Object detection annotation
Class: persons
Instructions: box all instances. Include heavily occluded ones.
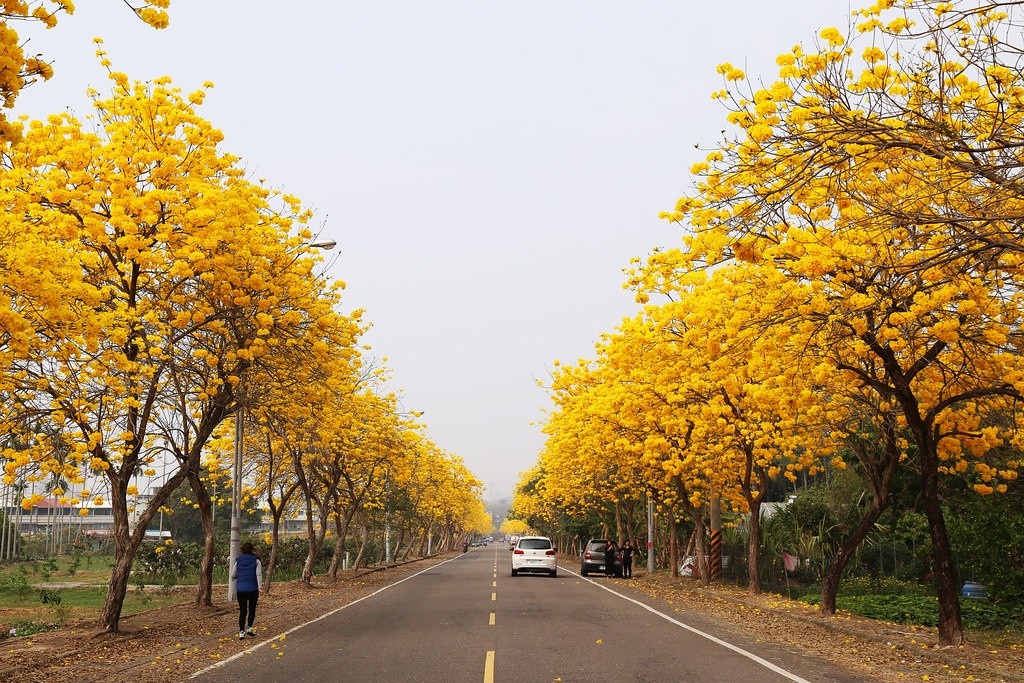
[605,540,615,578]
[464,539,468,548]
[231,542,263,640]
[618,539,635,579]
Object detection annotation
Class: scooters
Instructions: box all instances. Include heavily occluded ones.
[463,545,467,553]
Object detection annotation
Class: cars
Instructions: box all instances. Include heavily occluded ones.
[471,537,494,547]
[498,536,519,551]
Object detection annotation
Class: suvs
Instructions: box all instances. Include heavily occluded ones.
[509,535,559,579]
[578,538,623,578]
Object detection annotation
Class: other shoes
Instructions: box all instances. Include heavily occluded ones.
[239,631,246,640]
[246,627,255,636]
